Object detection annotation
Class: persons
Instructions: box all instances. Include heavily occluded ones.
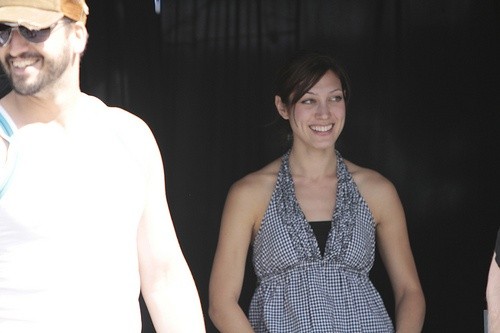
[485,223,500,333]
[0,0,206,333]
[211,57,426,333]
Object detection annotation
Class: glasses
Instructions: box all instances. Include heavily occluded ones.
[0,22,76,48]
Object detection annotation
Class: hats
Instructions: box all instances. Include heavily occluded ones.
[0,0,89,30]
